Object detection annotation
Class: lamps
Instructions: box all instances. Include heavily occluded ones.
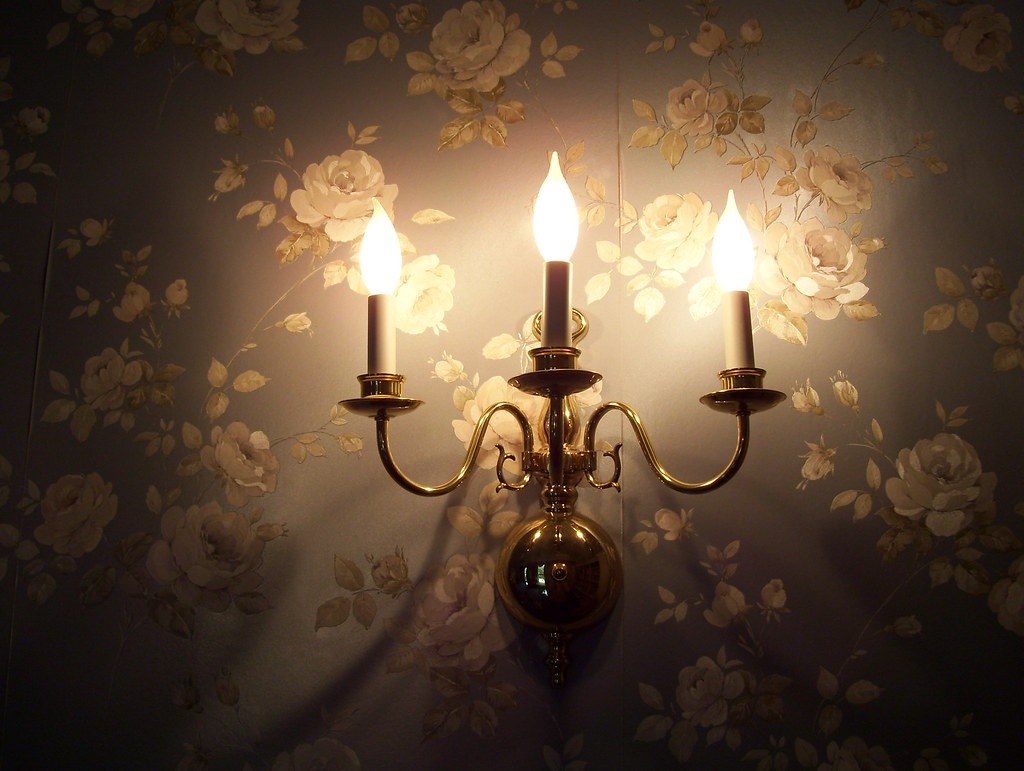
[336,151,786,690]
